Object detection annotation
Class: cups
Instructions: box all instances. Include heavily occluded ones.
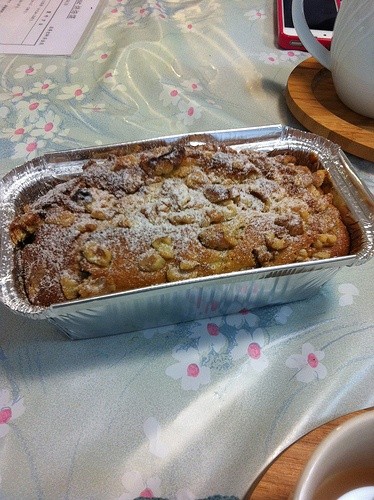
[291,0,374,119]
[291,410,374,500]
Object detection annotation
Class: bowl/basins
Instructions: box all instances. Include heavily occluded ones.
[0,124,374,339]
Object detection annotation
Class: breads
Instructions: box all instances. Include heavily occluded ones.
[10,134,351,305]
